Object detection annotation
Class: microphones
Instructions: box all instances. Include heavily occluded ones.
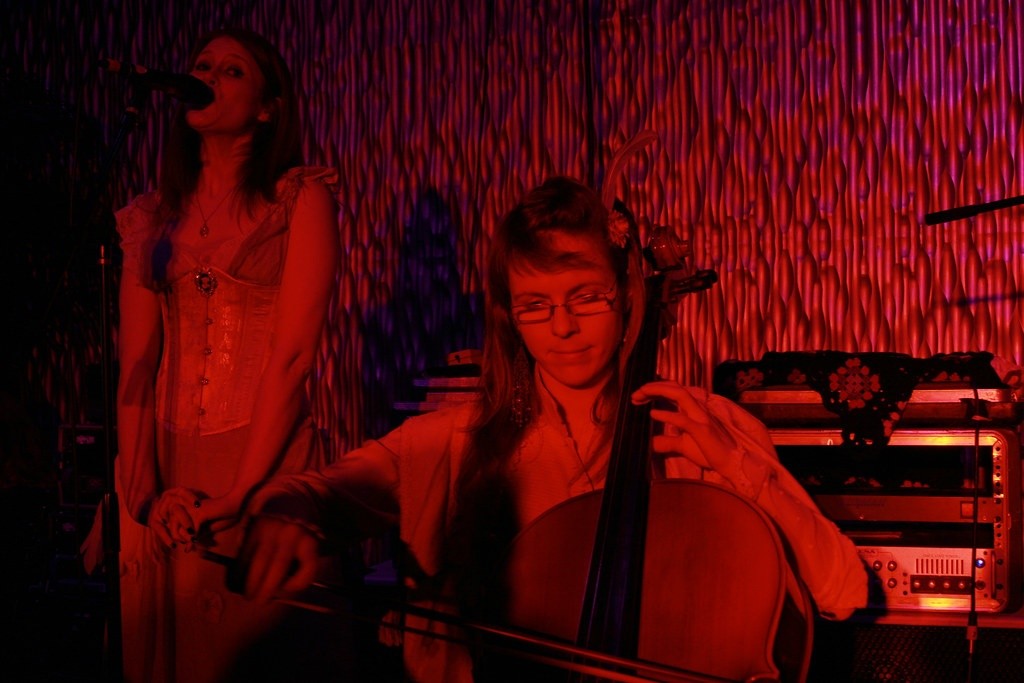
[103,59,214,109]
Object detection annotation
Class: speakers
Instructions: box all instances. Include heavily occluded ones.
[803,607,1024,683]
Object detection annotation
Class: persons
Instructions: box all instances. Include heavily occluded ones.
[237,178,887,682]
[112,32,346,683]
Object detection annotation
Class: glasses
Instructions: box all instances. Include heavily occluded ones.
[506,278,623,324]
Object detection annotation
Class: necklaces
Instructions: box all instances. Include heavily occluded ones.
[191,183,237,239]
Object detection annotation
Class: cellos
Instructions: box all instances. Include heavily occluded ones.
[502,199,813,683]
